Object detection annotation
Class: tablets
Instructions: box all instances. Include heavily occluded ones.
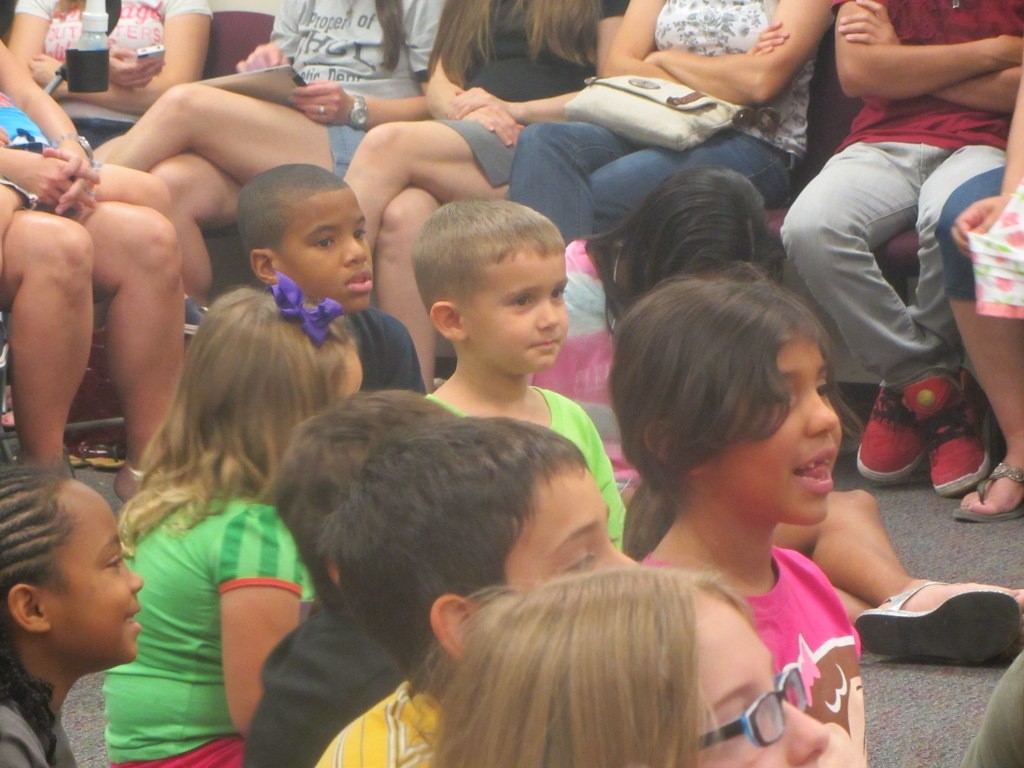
[196,65,308,112]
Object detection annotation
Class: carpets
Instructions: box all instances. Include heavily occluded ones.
[0,392,1023,767]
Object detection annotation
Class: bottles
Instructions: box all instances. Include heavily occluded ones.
[75,11,108,51]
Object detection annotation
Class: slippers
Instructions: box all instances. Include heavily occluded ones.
[952,462,1024,522]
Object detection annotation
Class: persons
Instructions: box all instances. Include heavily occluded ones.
[313,417,635,768]
[104,286,362,768]
[617,262,864,768]
[959,648,1024,768]
[239,391,453,768]
[0,468,145,768]
[430,565,829,768]
[0,0,1024,665]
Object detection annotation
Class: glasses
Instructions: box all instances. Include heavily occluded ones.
[700,662,809,750]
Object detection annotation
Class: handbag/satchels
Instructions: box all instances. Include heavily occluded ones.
[564,75,745,150]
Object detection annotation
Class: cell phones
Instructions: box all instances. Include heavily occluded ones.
[133,45,165,62]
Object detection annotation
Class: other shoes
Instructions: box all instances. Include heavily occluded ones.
[183,298,204,334]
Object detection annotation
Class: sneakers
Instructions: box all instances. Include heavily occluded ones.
[901,370,991,497]
[857,385,929,482]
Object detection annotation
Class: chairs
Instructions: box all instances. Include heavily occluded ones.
[764,202,925,305]
[0,286,200,477]
[205,9,275,80]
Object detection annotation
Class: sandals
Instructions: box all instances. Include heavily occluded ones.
[854,581,1020,661]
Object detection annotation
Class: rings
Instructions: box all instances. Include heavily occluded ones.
[320,106,323,113]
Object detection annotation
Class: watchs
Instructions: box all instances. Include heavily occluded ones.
[78,135,92,158]
[350,95,368,130]
